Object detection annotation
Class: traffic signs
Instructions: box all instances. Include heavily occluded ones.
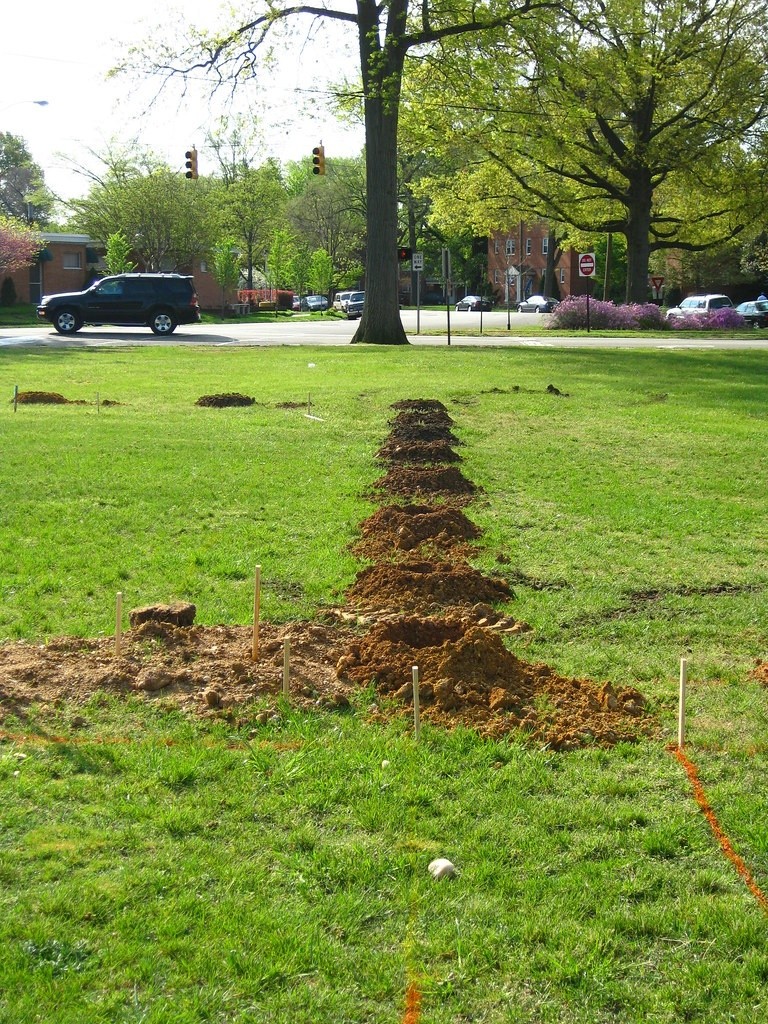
[413,254,424,271]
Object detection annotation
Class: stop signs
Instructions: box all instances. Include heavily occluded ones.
[578,253,596,277]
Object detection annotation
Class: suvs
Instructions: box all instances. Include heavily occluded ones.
[36,272,201,336]
[333,291,364,320]
[665,292,734,321]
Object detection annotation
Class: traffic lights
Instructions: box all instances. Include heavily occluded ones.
[312,139,326,176]
[398,246,411,260]
[184,142,199,180]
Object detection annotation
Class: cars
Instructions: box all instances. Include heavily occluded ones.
[455,295,492,312]
[293,296,300,311]
[301,295,328,312]
[735,301,768,329]
[517,295,560,314]
